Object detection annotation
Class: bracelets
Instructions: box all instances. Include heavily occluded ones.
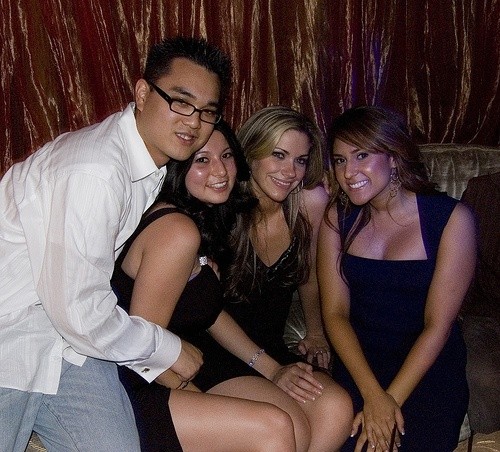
[177,381,188,390]
[249,349,264,367]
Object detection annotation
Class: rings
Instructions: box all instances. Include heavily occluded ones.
[316,350,323,355]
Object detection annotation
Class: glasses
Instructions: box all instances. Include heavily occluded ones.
[149,82,224,124]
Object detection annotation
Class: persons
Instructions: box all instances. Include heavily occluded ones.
[317,106,478,452]
[0,39,333,452]
[112,122,295,452]
[206,106,354,452]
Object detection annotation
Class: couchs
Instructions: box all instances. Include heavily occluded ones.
[283,143,500,452]
